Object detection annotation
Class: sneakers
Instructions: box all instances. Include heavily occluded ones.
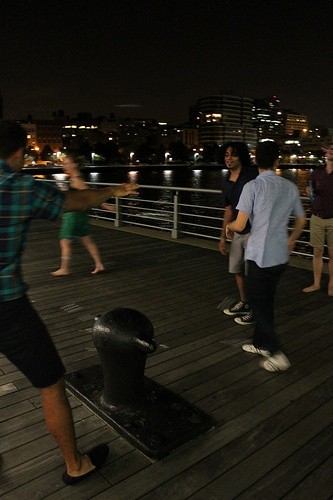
[223,301,250,316]
[264,353,291,372]
[242,344,271,357]
[235,312,256,325]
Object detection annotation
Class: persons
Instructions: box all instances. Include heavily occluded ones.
[218,142,259,326]
[0,117,139,483]
[225,140,307,373]
[49,156,105,277]
[301,144,333,298]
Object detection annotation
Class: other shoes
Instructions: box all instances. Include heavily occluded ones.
[62,443,109,485]
